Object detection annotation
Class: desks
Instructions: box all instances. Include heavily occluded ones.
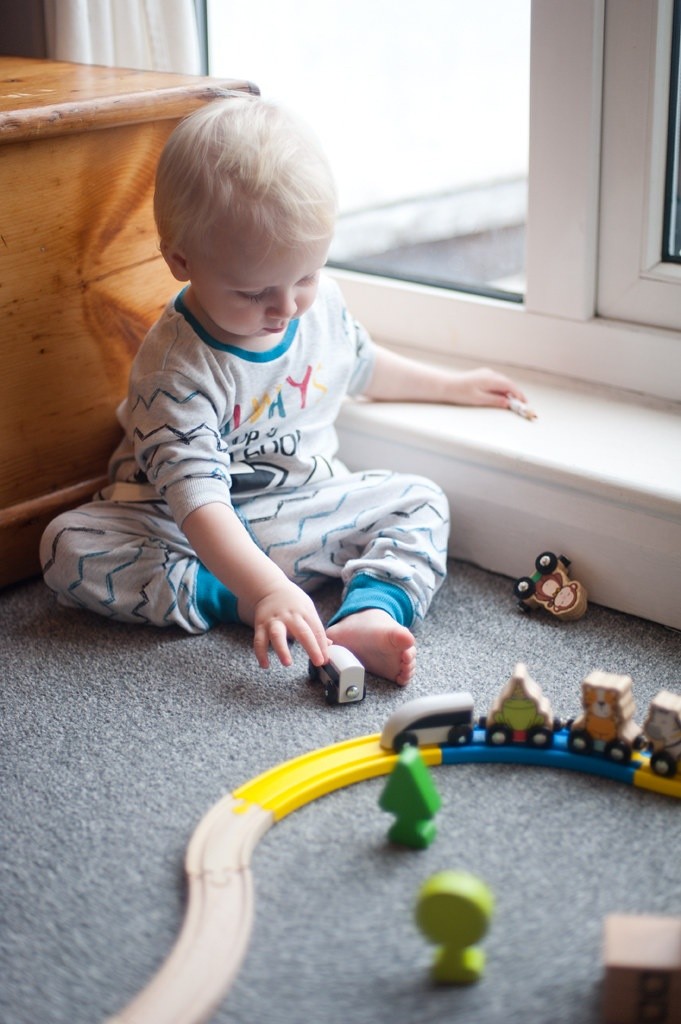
[0,49,259,582]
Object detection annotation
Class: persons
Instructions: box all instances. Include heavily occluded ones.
[37,82,530,689]
